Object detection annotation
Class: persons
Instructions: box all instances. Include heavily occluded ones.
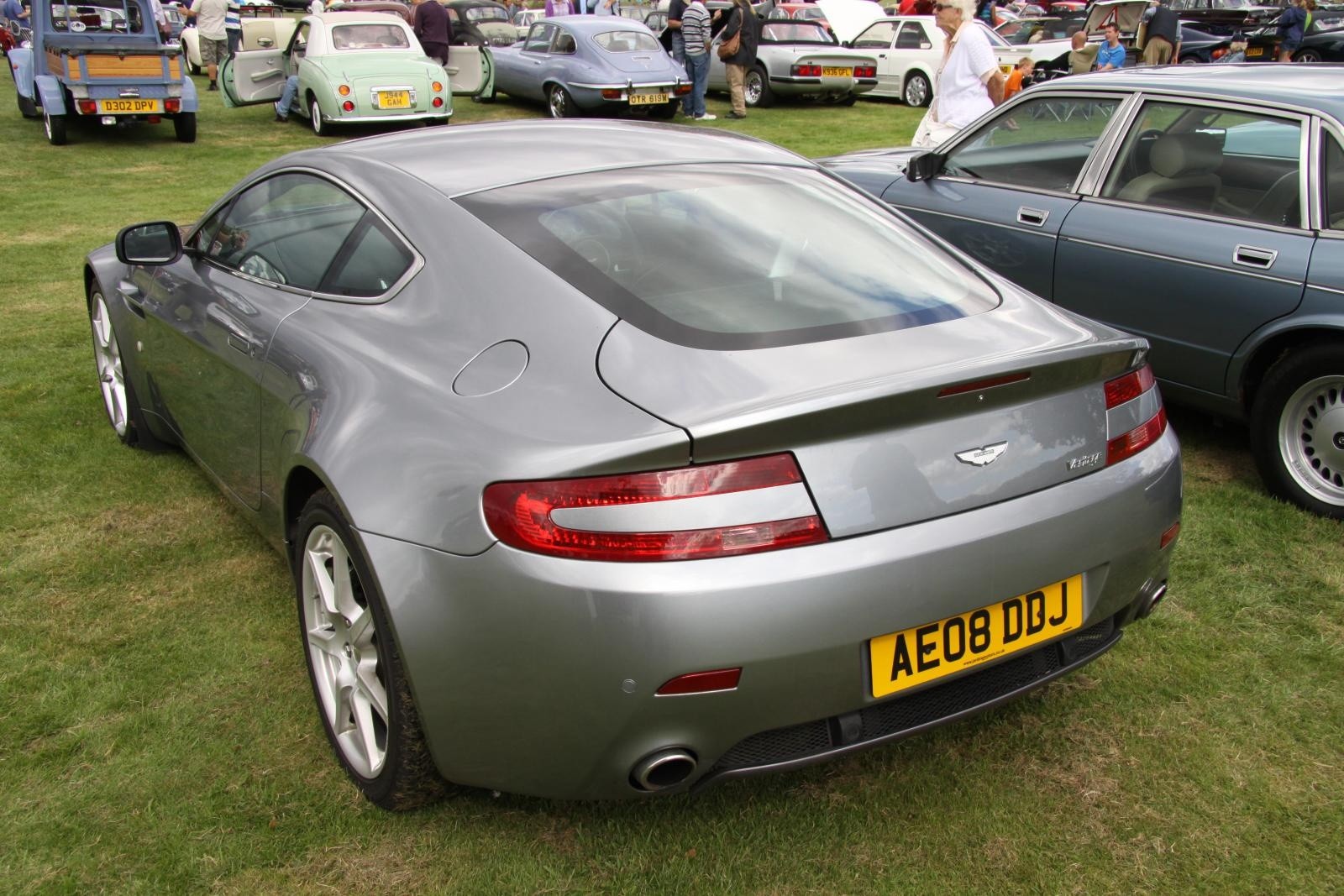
[1303,0,1317,33]
[501,0,534,25]
[1095,21,1125,71]
[587,0,617,15]
[1277,0,1311,62]
[414,0,454,67]
[545,0,575,40]
[273,75,298,121]
[1021,30,1087,117]
[975,0,997,29]
[911,0,1020,179]
[897,0,936,29]
[177,0,234,89]
[4,0,32,29]
[311,0,323,15]
[1142,1,1183,65]
[1211,33,1248,63]
[573,0,594,14]
[667,0,759,120]
[1004,57,1035,102]
[151,0,166,33]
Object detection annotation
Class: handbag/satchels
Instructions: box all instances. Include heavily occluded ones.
[910,101,959,150]
[717,8,744,56]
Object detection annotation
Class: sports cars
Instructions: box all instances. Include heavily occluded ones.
[80,116,1190,812]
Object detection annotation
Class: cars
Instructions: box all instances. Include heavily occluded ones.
[482,13,695,118]
[511,8,546,39]
[803,59,1343,527]
[156,0,523,138]
[617,6,669,31]
[637,0,1344,107]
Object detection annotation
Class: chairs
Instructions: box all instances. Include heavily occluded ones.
[1247,131,1344,228]
[557,34,572,49]
[1026,67,1116,123]
[1066,26,1081,37]
[897,32,921,49]
[377,35,400,46]
[112,19,141,33]
[341,30,370,47]
[607,40,628,52]
[1027,29,1053,45]
[1115,132,1222,214]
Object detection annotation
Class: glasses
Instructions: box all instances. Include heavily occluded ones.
[933,3,952,11]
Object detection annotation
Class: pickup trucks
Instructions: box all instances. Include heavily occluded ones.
[7,0,200,143]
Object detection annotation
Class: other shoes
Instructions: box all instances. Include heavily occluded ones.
[695,112,717,120]
[23,111,41,121]
[685,114,694,118]
[725,112,746,119]
[729,110,734,113]
[208,85,218,91]
[274,102,288,123]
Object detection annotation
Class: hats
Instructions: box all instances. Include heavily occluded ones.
[1231,33,1245,42]
